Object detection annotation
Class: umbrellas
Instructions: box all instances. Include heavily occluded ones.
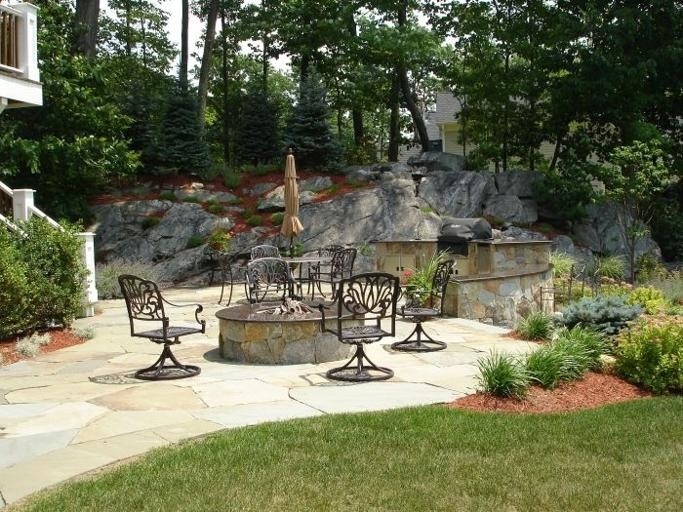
[279,146,304,260]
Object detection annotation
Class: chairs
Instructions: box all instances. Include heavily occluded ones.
[206,246,458,382]
[116,275,206,380]
